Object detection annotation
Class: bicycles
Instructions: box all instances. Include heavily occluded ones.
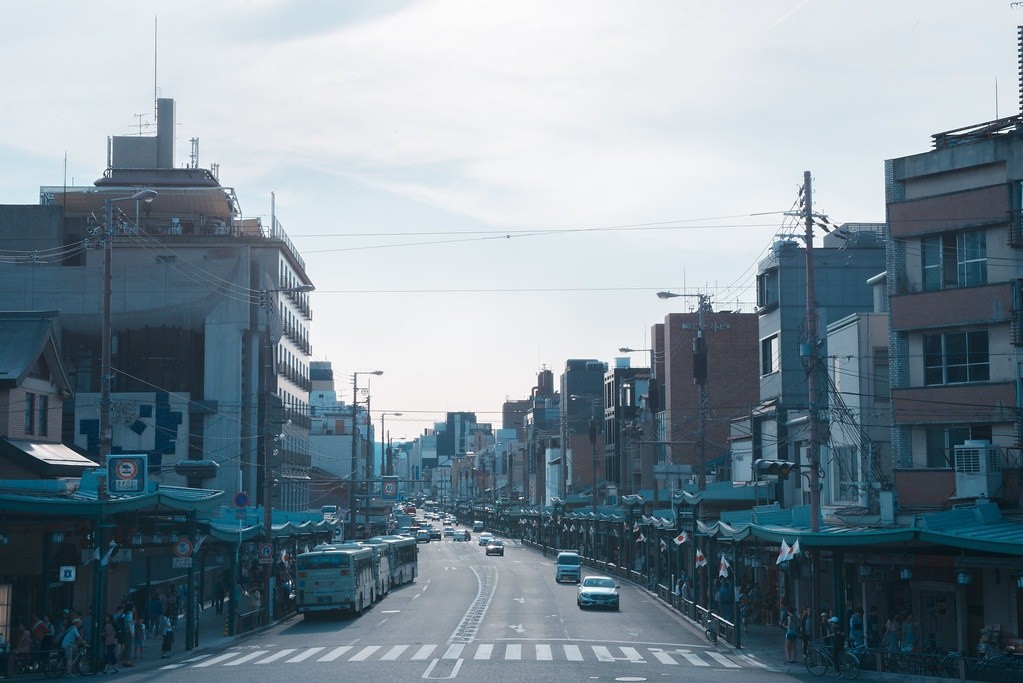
[805,636,861,680]
[702,610,718,646]
[43,643,102,679]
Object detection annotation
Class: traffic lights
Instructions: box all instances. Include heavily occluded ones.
[754,459,797,480]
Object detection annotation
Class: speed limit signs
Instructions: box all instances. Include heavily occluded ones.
[383,482,397,495]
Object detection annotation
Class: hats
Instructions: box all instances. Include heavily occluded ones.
[829,617,838,622]
[62,609,69,614]
[821,613,828,617]
[72,618,84,624]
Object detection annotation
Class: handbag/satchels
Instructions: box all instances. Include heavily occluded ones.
[58,635,65,648]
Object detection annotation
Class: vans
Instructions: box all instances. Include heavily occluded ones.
[554,552,583,584]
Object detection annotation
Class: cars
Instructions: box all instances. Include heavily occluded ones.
[401,502,505,556]
[577,576,620,611]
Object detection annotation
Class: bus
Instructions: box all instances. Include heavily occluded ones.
[295,535,420,621]
[423,501,438,513]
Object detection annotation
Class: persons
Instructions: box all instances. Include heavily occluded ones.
[713,576,773,632]
[101,583,181,674]
[0,608,87,677]
[676,569,693,603]
[215,561,291,627]
[819,609,844,679]
[849,607,865,648]
[633,555,662,591]
[580,542,584,557]
[881,609,915,671]
[866,605,881,649]
[783,606,810,664]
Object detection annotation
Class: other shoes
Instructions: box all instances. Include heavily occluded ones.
[66,672,76,678]
[114,668,119,672]
[784,661,791,665]
[835,673,843,679]
[29,666,33,670]
[34,664,39,669]
[790,660,796,664]
[101,670,107,674]
[161,655,171,659]
[123,663,135,667]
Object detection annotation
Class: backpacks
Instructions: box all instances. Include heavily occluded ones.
[118,612,132,632]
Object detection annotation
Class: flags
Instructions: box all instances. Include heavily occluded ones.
[695,549,707,568]
[624,522,645,542]
[562,524,594,534]
[304,546,309,552]
[673,530,689,545]
[281,550,288,568]
[719,557,730,578]
[776,538,800,565]
[192,535,207,553]
[519,517,562,528]
[83,548,100,567]
[660,540,666,552]
[101,547,114,566]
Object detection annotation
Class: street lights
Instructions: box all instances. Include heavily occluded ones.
[619,348,659,510]
[390,438,406,446]
[570,395,596,515]
[498,442,512,491]
[382,413,403,476]
[523,427,540,503]
[351,371,383,539]
[657,292,707,491]
[262,284,314,624]
[89,190,158,672]
